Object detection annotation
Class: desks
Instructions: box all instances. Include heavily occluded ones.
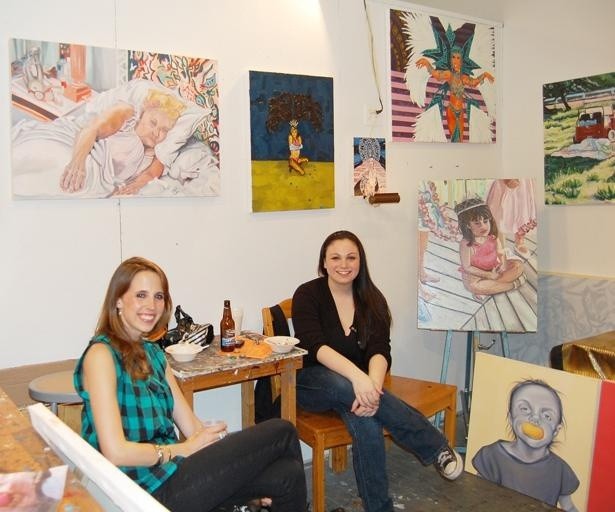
[560,331,615,382]
[27,367,81,415]
[164,329,304,441]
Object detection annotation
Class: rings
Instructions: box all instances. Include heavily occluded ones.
[219,433,226,440]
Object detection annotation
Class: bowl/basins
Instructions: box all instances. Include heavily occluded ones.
[264,335,300,353]
[164,343,203,362]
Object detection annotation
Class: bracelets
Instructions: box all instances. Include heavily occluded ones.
[166,446,172,462]
[154,444,164,468]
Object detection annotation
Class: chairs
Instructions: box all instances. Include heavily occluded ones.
[382,369,456,453]
[260,296,386,511]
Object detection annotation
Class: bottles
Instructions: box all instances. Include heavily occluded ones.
[219,300,236,351]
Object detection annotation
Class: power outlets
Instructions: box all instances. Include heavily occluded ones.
[364,105,381,125]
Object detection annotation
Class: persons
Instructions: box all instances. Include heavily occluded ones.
[60,89,188,196]
[453,198,528,302]
[418,182,458,301]
[471,380,579,512]
[288,126,308,175]
[291,231,463,512]
[487,179,537,253]
[72,257,343,512]
[416,46,495,142]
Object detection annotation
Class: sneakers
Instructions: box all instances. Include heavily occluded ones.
[434,444,464,480]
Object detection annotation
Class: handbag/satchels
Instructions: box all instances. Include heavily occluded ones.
[254,375,281,422]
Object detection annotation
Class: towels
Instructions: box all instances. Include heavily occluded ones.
[216,335,273,359]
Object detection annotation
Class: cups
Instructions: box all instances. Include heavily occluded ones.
[232,308,243,337]
